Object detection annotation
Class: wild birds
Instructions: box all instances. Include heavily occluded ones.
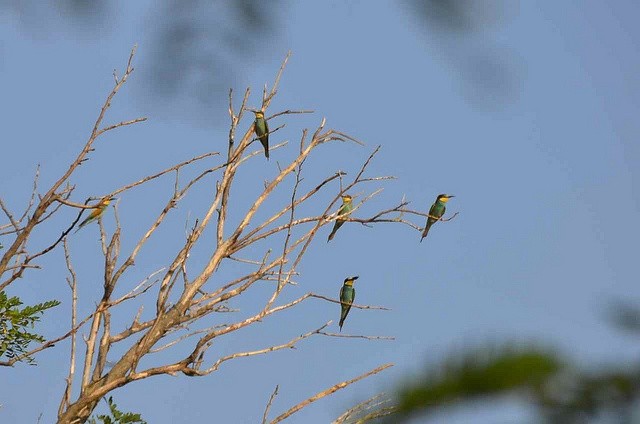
[327,195,352,243]
[251,110,270,160]
[339,276,358,332]
[74,196,117,235]
[420,193,456,243]
